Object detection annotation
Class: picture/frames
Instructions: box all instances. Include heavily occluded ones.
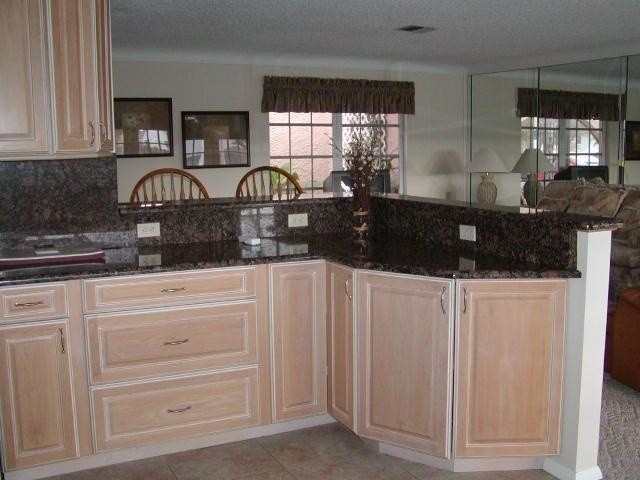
[181,110,251,169]
[113,97,174,158]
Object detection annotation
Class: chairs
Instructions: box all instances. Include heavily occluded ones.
[236,165,303,198]
[130,168,209,203]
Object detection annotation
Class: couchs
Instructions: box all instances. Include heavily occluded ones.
[535,181,640,392]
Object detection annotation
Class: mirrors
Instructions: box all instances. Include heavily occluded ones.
[467,54,640,209]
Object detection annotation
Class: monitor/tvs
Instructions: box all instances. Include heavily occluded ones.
[554,165,609,184]
[323,169,391,193]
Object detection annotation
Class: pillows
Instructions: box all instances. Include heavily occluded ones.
[533,181,640,245]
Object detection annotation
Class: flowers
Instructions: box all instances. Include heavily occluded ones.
[322,109,398,234]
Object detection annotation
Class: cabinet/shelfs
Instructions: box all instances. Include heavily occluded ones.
[357,269,567,459]
[270,261,328,423]
[1,283,74,473]
[329,263,357,436]
[0,0,116,163]
[81,265,261,455]
